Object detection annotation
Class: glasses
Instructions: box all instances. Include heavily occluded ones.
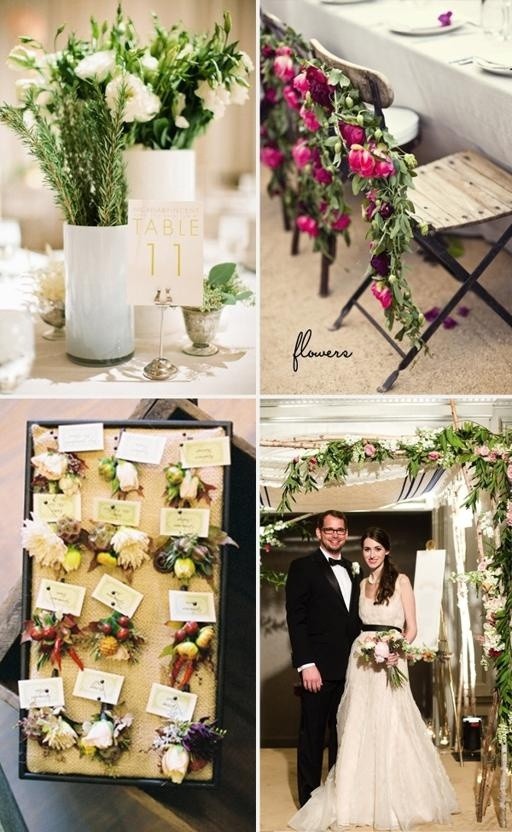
[324,528,346,535]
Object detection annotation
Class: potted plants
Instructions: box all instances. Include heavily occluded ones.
[180,262,253,358]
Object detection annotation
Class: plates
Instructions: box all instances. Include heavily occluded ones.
[320,0,512,81]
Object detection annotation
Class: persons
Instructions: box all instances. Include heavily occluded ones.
[286,510,364,808]
[345,527,418,831]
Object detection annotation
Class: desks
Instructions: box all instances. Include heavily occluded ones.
[261,1,511,252]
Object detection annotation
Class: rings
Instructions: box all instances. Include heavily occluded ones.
[394,662,396,666]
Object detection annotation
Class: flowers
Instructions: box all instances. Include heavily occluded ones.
[355,626,410,688]
[65,5,257,149]
[2,29,159,226]
[260,511,290,552]
[277,419,511,724]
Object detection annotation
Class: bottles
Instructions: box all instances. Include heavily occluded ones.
[0,216,38,393]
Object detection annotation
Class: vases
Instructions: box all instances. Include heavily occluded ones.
[121,143,194,311]
[61,221,136,366]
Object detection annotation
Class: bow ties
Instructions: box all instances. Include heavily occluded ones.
[329,558,347,568]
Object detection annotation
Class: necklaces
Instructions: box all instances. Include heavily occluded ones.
[368,573,382,585]
